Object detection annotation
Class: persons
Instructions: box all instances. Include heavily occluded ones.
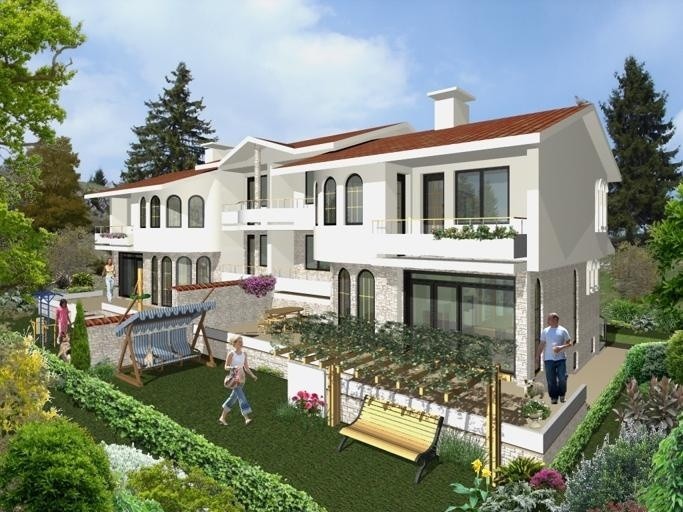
[101,257,116,303]
[53,299,72,345]
[218,334,258,427]
[535,312,573,404]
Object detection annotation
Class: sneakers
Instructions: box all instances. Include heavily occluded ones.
[218,418,229,426]
[560,395,566,403]
[245,418,254,426]
[551,395,558,404]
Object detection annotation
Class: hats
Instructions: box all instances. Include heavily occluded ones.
[227,333,241,346]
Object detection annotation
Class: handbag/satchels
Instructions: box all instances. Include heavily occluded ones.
[222,367,241,390]
